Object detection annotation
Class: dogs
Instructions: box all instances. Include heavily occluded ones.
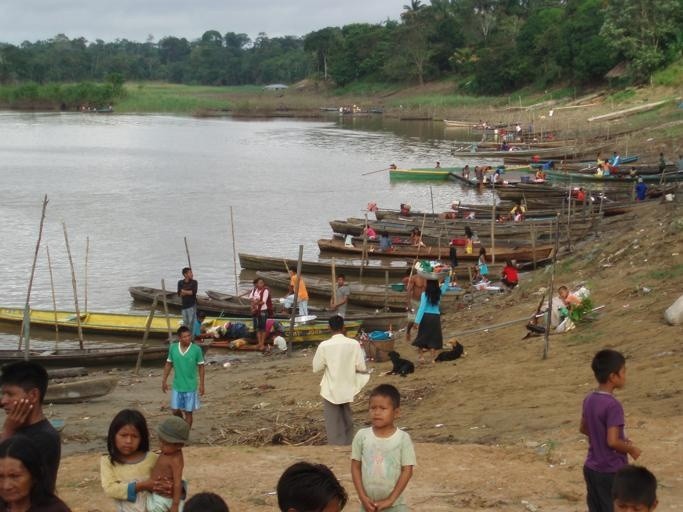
[435,340,463,362]
[386,351,415,377]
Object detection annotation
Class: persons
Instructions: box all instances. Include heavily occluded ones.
[277,461,348,512]
[462,225,518,291]
[658,152,665,172]
[270,332,288,354]
[611,464,657,512]
[178,267,198,332]
[535,161,556,180]
[463,165,503,184]
[363,202,423,251]
[559,285,592,322]
[288,266,309,316]
[330,275,350,320]
[101,409,188,512]
[183,492,229,512]
[312,315,370,445]
[479,120,553,151]
[162,326,205,429]
[248,278,271,351]
[578,187,586,199]
[511,201,525,224]
[403,268,450,362]
[596,152,618,175]
[146,416,190,512]
[351,384,416,512]
[0,361,61,479]
[0,434,72,512]
[578,350,641,512]
[630,167,647,201]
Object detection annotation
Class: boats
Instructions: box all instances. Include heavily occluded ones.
[204,290,408,330]
[127,286,403,332]
[237,252,516,279]
[0,343,209,366]
[255,271,466,311]
[80,109,113,114]
[319,108,382,114]
[42,376,119,404]
[0,307,363,343]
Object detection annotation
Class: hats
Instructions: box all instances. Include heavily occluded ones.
[153,416,190,446]
